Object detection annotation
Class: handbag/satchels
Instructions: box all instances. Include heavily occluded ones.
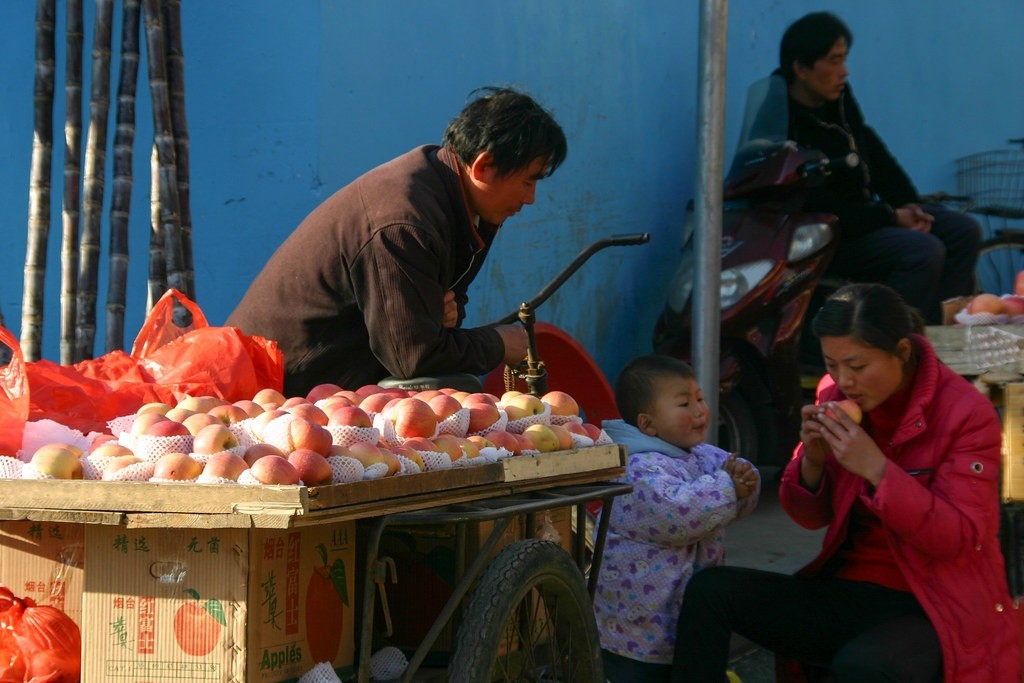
[0,326,30,457]
[131,288,285,403]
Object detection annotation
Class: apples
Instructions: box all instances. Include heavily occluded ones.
[0,599,83,683]
[966,269,1024,317]
[825,399,863,426]
[28,384,602,487]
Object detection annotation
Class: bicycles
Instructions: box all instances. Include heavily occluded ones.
[945,137,1024,296]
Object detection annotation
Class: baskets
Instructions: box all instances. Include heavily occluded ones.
[954,149,1024,217]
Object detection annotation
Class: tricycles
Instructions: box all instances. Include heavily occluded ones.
[0,232,652,683]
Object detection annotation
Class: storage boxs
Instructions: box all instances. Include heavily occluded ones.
[0,520,85,630]
[988,382,1024,504]
[355,506,572,658]
[80,520,357,683]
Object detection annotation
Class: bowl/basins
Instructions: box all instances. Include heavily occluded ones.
[481,321,621,517]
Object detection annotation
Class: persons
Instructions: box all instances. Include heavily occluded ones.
[225,86,569,398]
[775,281,1024,683]
[591,355,760,683]
[751,11,982,324]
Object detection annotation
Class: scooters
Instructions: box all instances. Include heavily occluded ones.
[650,71,917,498]
